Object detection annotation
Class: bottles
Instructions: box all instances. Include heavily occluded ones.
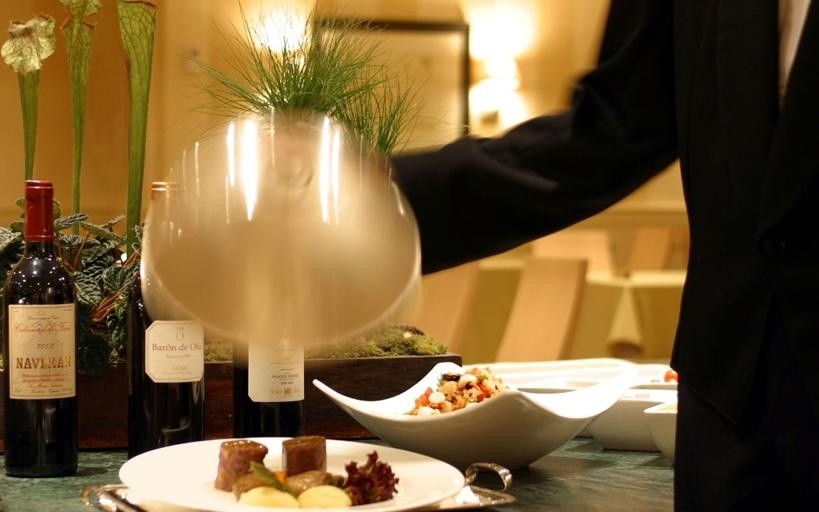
[2,179,82,479]
[230,334,308,439]
[126,179,206,461]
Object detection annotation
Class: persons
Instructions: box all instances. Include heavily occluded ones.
[387,0,818,512]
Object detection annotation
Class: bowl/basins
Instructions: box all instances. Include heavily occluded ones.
[310,359,635,473]
[520,384,678,463]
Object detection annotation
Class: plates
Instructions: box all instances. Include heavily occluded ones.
[115,434,471,512]
